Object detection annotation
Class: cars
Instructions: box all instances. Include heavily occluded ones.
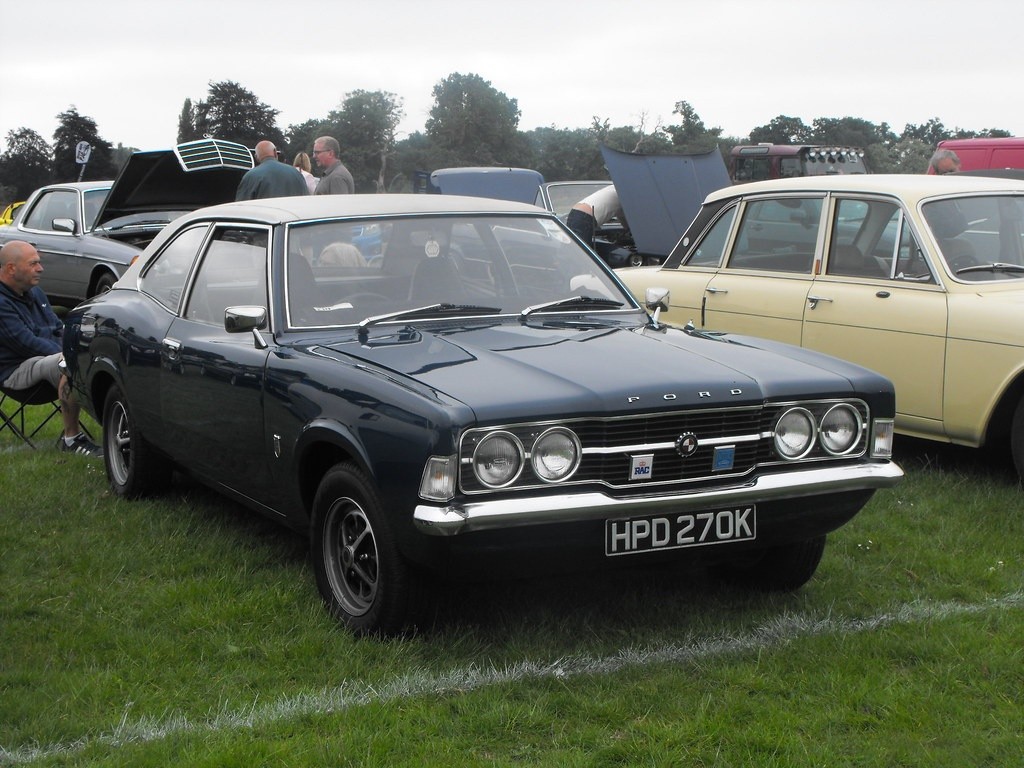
[925,135,1024,262]
[1,139,254,310]
[62,195,907,637]
[596,140,1024,483]
[426,161,639,267]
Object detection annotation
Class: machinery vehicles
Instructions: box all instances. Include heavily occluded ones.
[727,134,916,261]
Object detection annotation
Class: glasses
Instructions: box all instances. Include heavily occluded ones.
[313,150,331,155]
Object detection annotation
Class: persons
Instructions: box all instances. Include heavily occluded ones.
[0,240,105,459]
[318,243,367,267]
[313,136,354,259]
[292,152,316,195]
[235,140,311,265]
[931,150,962,175]
[567,185,629,248]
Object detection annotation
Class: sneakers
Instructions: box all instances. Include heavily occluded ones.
[62,432,104,458]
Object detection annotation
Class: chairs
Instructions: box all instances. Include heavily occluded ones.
[828,244,879,278]
[261,252,339,329]
[407,253,473,311]
[0,378,96,451]
[936,236,980,282]
